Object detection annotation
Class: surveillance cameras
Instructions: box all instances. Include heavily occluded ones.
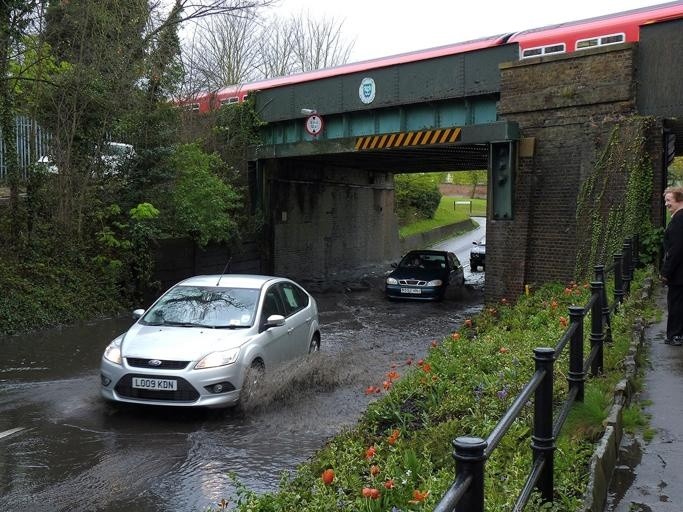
[301,109,316,115]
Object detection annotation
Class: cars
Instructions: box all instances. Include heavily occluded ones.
[97,272,325,415]
[383,248,466,302]
[48,139,140,181]
[468,233,486,272]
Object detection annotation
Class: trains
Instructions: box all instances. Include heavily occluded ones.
[170,0,683,120]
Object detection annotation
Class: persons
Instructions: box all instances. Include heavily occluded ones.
[657,185,683,348]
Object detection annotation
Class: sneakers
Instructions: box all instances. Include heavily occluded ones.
[665,336,683,345]
